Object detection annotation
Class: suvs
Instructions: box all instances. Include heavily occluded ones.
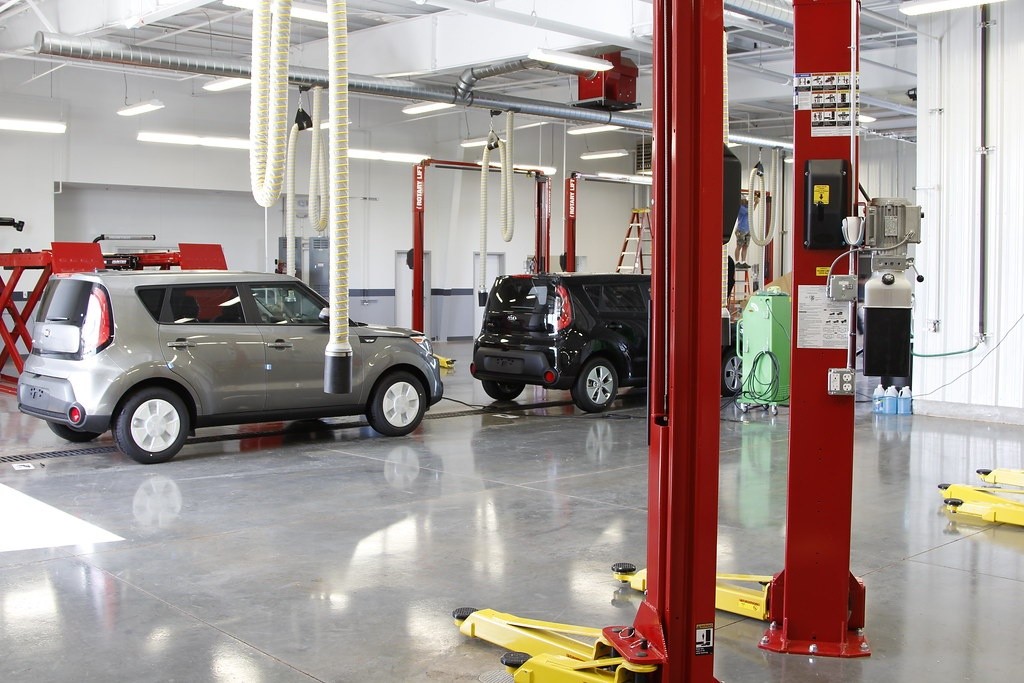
[16,269,444,464]
[469,271,742,412]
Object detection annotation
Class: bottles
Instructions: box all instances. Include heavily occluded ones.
[872,384,912,414]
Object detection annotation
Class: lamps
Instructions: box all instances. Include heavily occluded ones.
[727,131,796,153]
[480,161,555,175]
[0,54,434,171]
[599,173,652,186]
[567,124,623,135]
[224,0,330,24]
[462,136,499,148]
[403,100,453,115]
[528,48,612,72]
[581,149,628,159]
[897,0,1006,17]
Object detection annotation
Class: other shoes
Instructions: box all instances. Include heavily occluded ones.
[743,263,751,268]
[736,262,743,268]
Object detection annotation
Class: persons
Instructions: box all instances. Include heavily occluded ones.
[735,194,761,269]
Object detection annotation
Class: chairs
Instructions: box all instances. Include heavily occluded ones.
[183,294,199,324]
[216,299,245,323]
